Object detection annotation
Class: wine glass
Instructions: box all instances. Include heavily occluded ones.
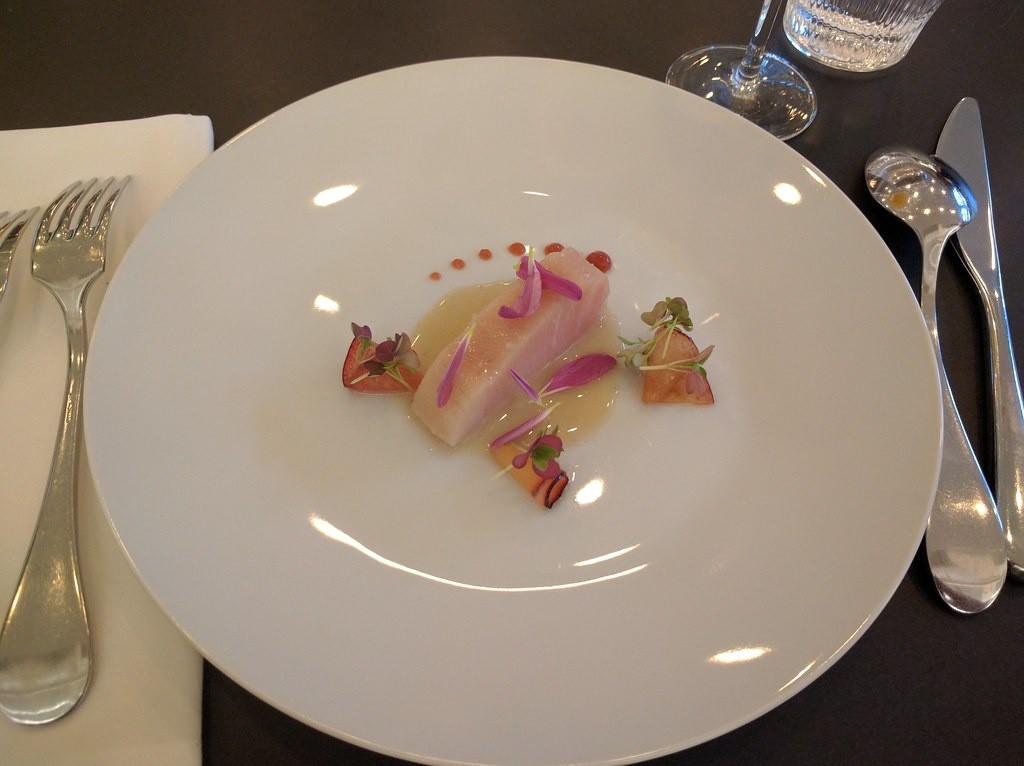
[665,0,818,141]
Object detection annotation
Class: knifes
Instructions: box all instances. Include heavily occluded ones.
[936,96,1024,580]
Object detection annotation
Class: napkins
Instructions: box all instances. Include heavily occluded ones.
[0,110,216,766]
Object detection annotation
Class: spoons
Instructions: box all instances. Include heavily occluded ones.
[865,143,1009,614]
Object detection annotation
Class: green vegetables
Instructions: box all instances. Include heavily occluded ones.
[351,245,714,482]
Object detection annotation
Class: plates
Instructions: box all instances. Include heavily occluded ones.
[86,55,942,766]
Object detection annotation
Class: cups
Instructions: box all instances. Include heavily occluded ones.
[782,0,944,73]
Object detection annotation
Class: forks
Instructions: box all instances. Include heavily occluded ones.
[0,176,131,725]
[0,206,40,302]
